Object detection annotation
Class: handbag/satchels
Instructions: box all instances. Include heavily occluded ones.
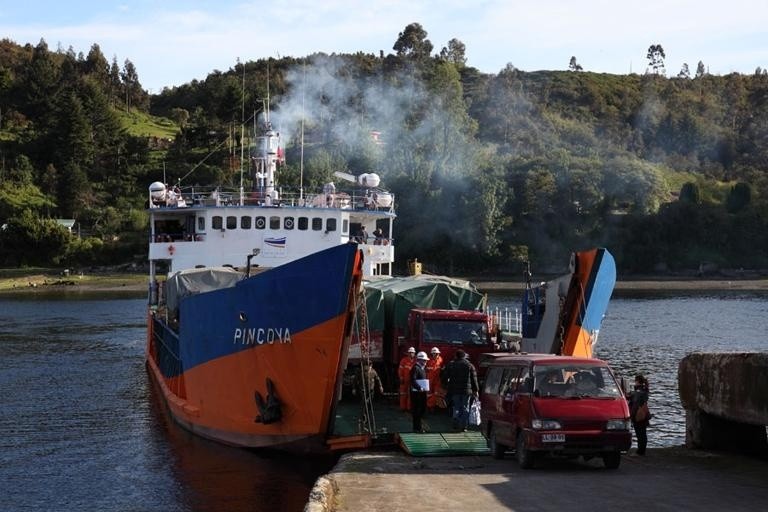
[468,401,482,425]
[635,402,649,422]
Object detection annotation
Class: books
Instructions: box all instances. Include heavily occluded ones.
[412,378,430,392]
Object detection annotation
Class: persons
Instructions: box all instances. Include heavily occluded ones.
[408,350,431,432]
[442,348,480,431]
[352,359,384,425]
[355,225,369,243]
[425,346,445,412]
[624,374,652,456]
[322,177,336,208]
[490,323,503,345]
[397,347,417,417]
[155,229,195,242]
[372,228,385,245]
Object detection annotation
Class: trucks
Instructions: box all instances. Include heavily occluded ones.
[353,272,497,402]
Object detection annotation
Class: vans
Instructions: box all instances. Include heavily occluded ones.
[479,353,633,473]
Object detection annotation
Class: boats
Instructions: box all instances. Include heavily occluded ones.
[146,59,618,450]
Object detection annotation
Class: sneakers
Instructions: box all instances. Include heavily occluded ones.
[453,422,471,432]
[430,409,447,415]
[399,409,413,414]
[416,429,426,433]
[630,451,646,457]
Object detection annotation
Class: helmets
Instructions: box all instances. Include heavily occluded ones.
[417,352,430,360]
[430,347,441,353]
[408,347,416,352]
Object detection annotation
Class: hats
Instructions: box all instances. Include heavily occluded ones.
[365,360,372,366]
[374,228,385,238]
[456,351,465,357]
